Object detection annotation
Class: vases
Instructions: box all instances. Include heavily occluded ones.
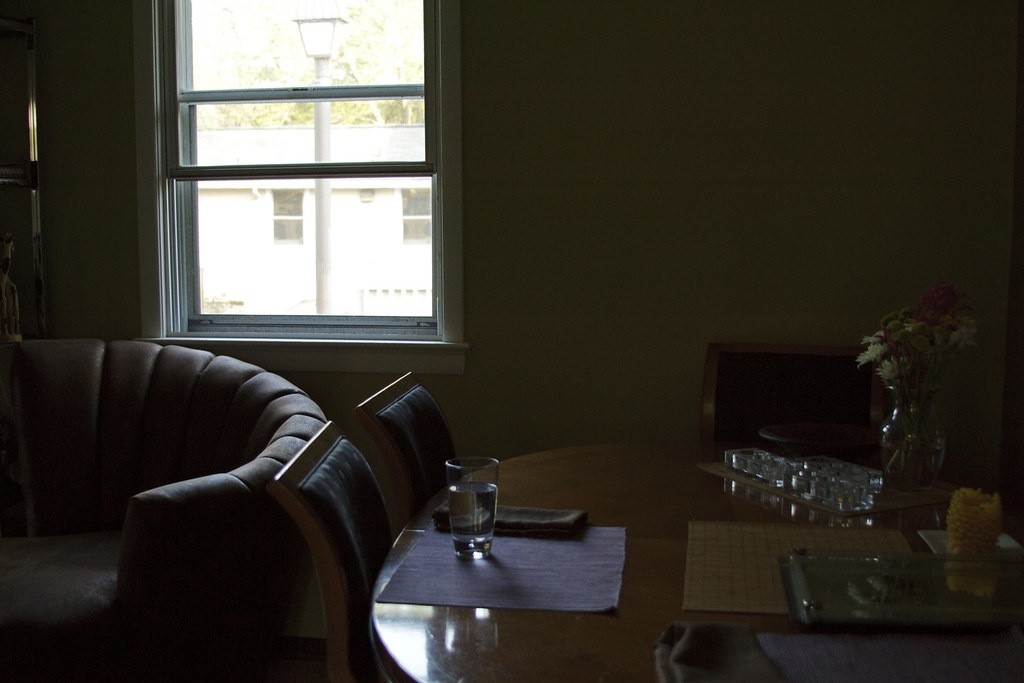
[880,400,942,492]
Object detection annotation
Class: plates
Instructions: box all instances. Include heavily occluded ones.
[916,528,1024,554]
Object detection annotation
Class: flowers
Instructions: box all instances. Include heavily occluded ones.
[857,279,977,469]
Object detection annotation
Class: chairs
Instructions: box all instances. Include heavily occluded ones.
[352,372,462,525]
[267,420,392,683]
[698,343,885,441]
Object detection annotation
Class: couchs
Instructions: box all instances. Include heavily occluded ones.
[0,337,328,683]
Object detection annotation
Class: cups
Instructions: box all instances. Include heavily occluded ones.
[445,456,500,560]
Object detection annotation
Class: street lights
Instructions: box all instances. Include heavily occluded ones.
[291,0,351,315]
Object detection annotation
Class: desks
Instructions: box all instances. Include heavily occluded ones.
[368,435,1024,683]
